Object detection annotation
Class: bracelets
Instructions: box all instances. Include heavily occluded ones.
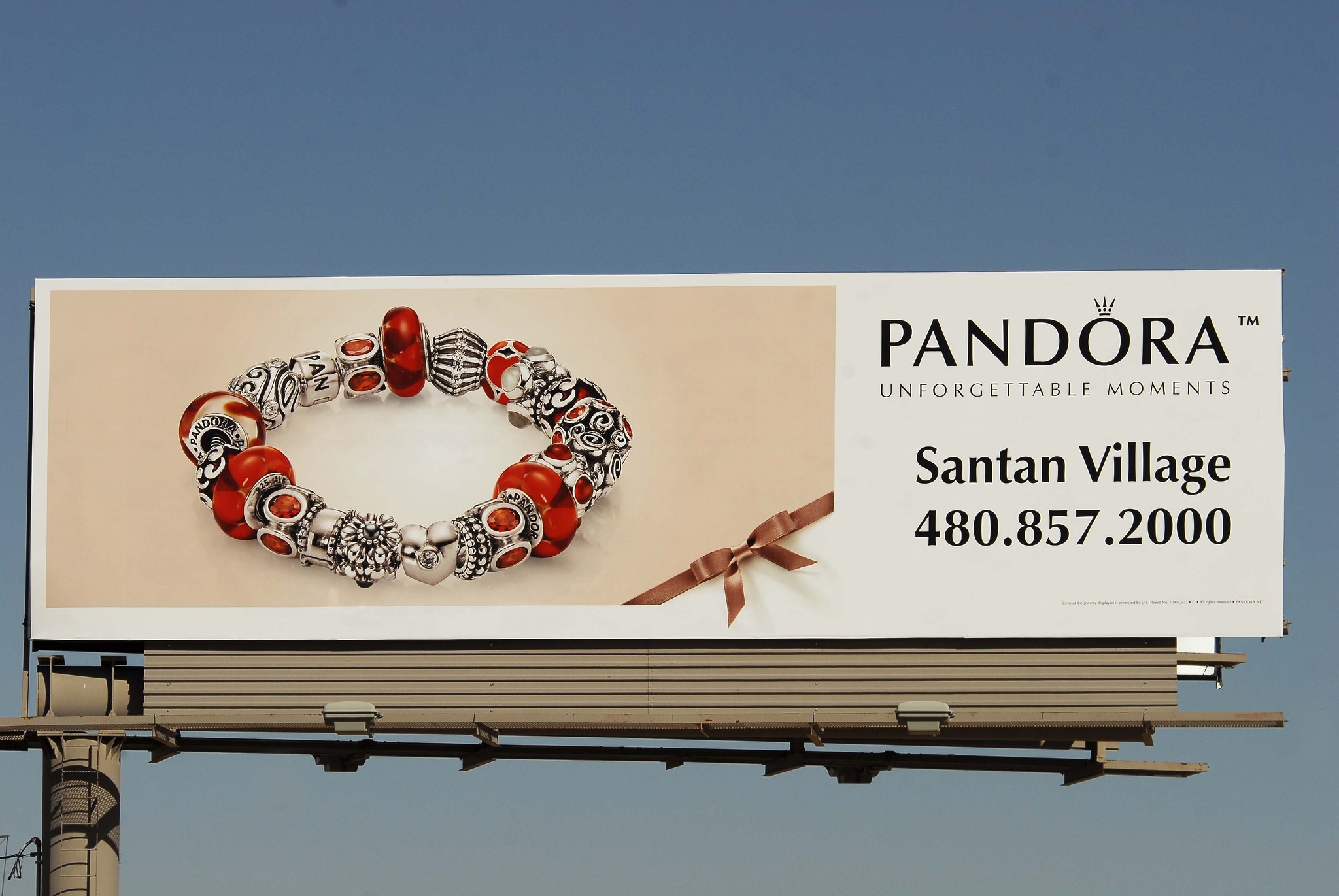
[179,307,634,589]
